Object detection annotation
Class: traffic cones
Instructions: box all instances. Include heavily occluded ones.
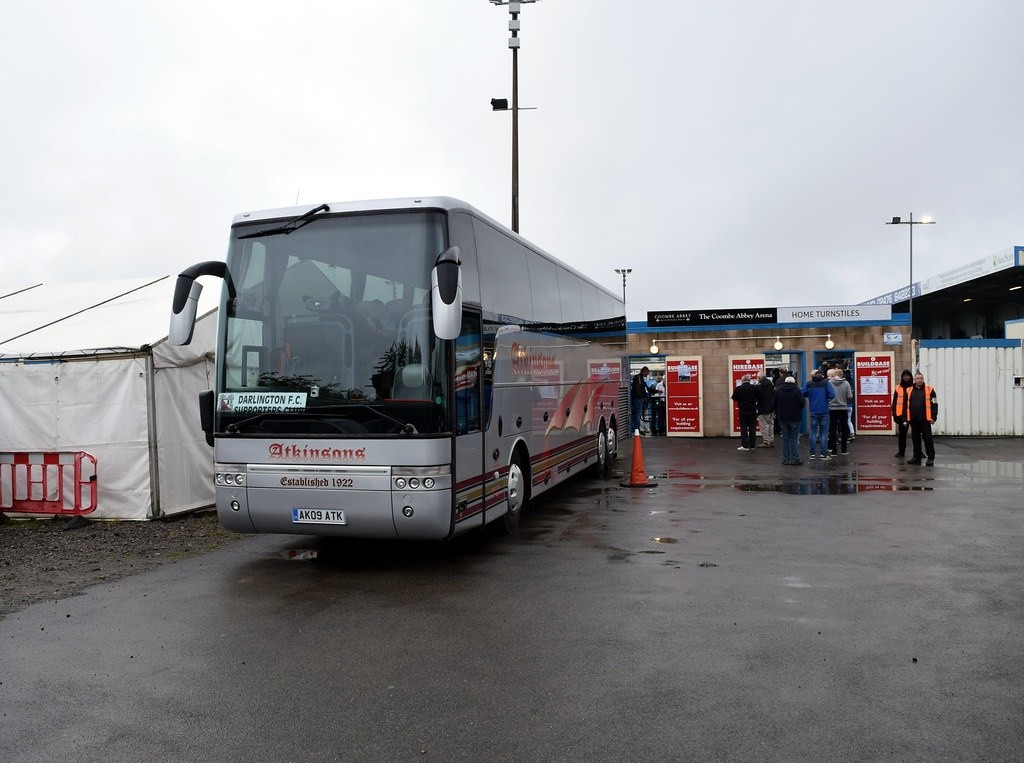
[617,427,660,490]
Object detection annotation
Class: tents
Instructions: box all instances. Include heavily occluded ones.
[0,275,224,519]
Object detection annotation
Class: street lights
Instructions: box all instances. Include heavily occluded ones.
[488,0,542,234]
[884,212,939,339]
[614,268,632,304]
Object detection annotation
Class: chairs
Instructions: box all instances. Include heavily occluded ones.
[318,288,432,401]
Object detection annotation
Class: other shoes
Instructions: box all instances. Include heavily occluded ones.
[895,452,905,457]
[639,431,646,435]
[770,444,775,448]
[926,459,934,466]
[758,443,769,448]
[907,457,921,465]
[922,453,926,458]
[841,450,849,455]
[838,434,855,444]
[830,453,836,457]
[782,460,790,465]
[737,446,750,450]
[791,460,805,465]
[827,448,832,452]
[809,454,816,460]
[751,447,756,451]
[820,455,832,461]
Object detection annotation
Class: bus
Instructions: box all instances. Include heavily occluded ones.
[165,196,633,552]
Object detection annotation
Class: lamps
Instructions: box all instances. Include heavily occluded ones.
[825,333,834,350]
[774,336,783,350]
[649,340,658,354]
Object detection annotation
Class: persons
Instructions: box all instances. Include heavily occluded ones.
[771,368,806,464]
[731,375,756,451]
[802,358,856,460]
[755,372,775,447]
[632,366,663,434]
[892,370,926,458]
[648,374,666,436]
[903,373,938,466]
